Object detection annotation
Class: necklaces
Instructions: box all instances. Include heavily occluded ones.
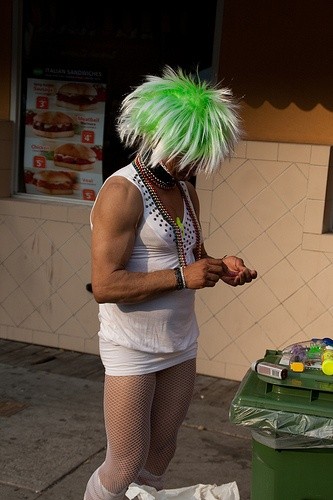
[135,145,202,269]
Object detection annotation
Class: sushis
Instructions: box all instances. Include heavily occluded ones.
[32,111,75,139]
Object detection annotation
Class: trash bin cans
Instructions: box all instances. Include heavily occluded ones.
[227,350,332,500]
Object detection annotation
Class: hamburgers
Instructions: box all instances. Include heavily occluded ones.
[55,83,99,111]
[53,143,96,171]
[32,171,81,195]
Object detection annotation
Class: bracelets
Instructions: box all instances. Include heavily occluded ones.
[173,267,183,290]
[178,266,185,289]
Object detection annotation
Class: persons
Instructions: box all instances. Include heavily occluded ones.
[80,67,259,500]
